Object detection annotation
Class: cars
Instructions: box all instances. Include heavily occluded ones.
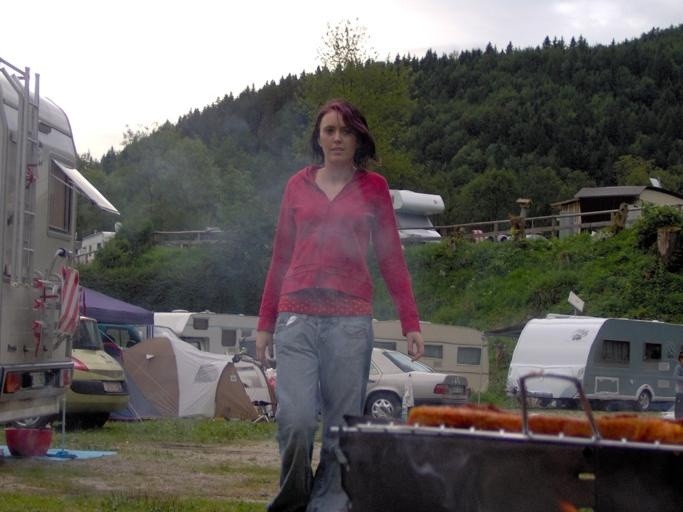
[259,347,470,425]
[504,233,547,243]
[52,312,130,433]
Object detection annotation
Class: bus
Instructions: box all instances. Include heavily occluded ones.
[147,228,222,251]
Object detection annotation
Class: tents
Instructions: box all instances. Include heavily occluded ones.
[108,337,277,422]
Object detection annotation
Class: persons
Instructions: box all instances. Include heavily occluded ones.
[256,98,424,512]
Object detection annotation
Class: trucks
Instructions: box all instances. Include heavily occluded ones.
[0,56,119,456]
[152,309,490,398]
[370,188,444,250]
[505,309,682,414]
[70,232,131,265]
[97,321,201,354]
